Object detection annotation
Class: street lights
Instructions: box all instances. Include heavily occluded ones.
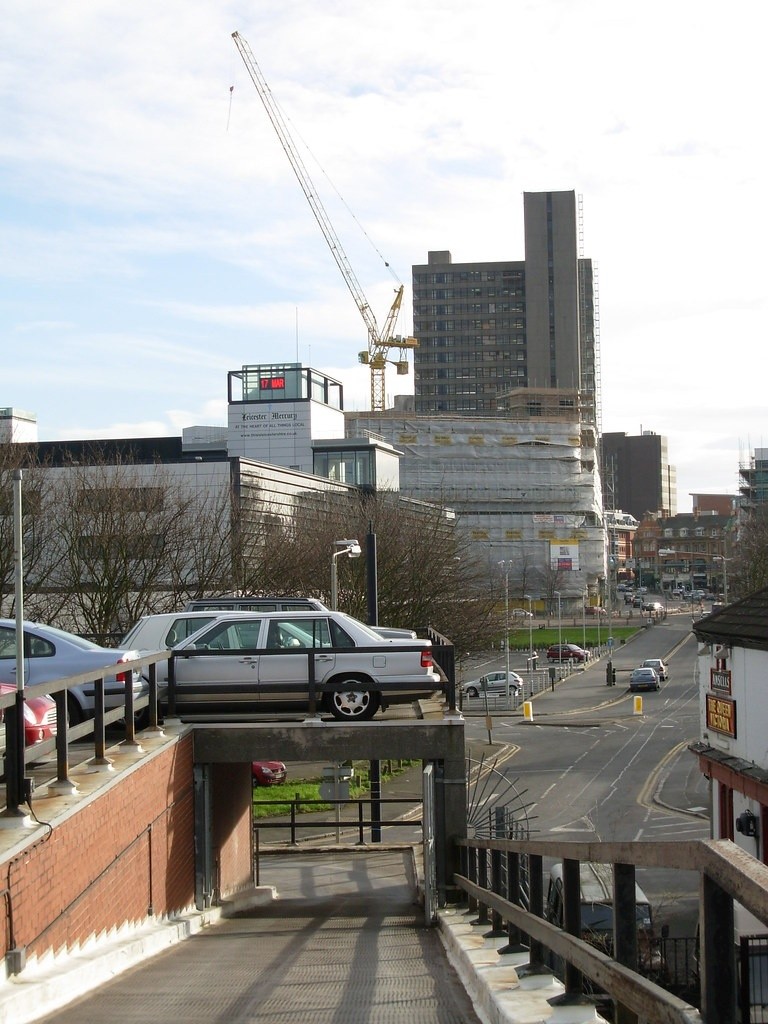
[578,588,585,665]
[590,583,600,656]
[523,594,532,681]
[331,544,361,611]
[497,559,513,707]
[712,556,749,596]
[659,548,727,608]
[333,538,360,611]
[554,590,561,666]
[603,582,612,653]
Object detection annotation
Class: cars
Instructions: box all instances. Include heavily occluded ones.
[617,584,664,611]
[0,682,57,784]
[462,671,524,698]
[119,611,332,651]
[252,761,287,788]
[629,667,660,692]
[138,611,441,722]
[508,608,533,617]
[640,658,669,681]
[585,606,607,616]
[672,589,740,602]
[0,619,143,744]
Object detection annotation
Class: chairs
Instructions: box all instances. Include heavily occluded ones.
[268,626,282,651]
[33,641,45,656]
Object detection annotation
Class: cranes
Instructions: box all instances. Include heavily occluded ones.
[232,31,421,412]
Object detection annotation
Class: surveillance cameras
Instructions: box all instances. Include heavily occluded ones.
[422,765,432,777]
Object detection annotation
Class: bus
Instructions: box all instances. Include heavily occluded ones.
[548,863,670,981]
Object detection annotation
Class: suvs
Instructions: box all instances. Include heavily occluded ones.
[182,598,417,638]
[547,644,593,663]
